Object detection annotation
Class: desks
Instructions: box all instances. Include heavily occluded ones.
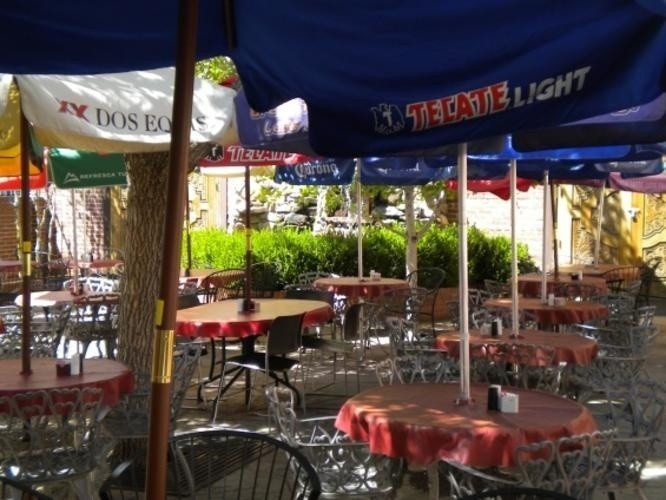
[4,358,135,414]
[336,383,599,470]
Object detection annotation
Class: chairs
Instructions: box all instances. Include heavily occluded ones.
[438,428,619,500]
[4,386,106,497]
[267,385,396,500]
[600,376,665,497]
[166,429,323,500]
[209,310,306,420]
[105,344,201,497]
[1,244,665,377]
